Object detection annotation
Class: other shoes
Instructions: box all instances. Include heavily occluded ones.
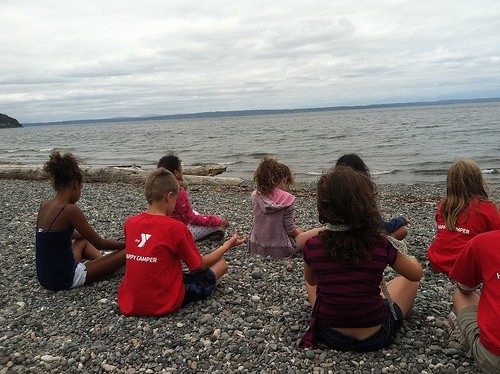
[197,230,225,242]
[216,277,222,286]
[447,312,466,344]
[113,265,126,276]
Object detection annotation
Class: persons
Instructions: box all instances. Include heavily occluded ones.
[426,159,500,279]
[318,154,409,253]
[149,155,230,244]
[246,159,319,258]
[35,151,137,291]
[117,167,246,318]
[298,165,424,353]
[448,229,500,374]
